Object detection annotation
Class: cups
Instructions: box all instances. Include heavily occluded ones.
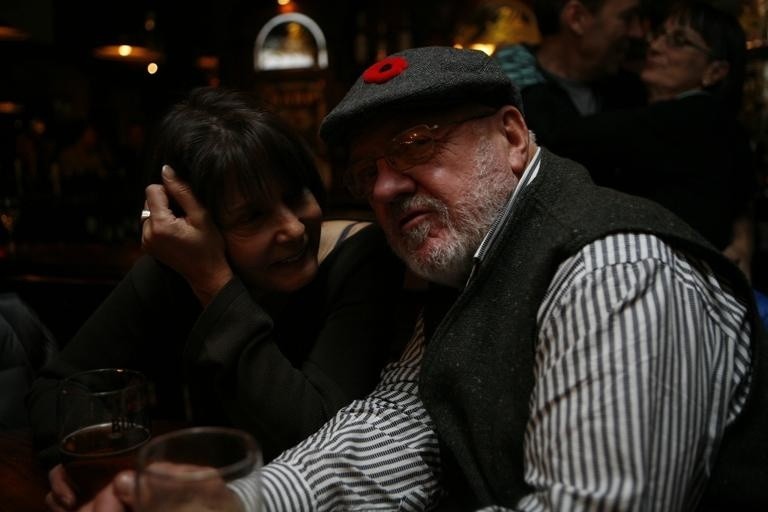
[134,427,263,510]
[54,368,146,512]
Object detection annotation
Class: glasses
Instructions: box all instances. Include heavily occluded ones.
[646,29,714,56]
[343,105,502,199]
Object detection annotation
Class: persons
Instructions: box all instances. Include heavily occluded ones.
[556,2,745,260]
[22,81,414,511]
[76,44,766,509]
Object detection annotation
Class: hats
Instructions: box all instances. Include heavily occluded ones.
[318,45,525,153]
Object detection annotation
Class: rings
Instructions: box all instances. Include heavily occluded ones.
[140,208,151,224]
